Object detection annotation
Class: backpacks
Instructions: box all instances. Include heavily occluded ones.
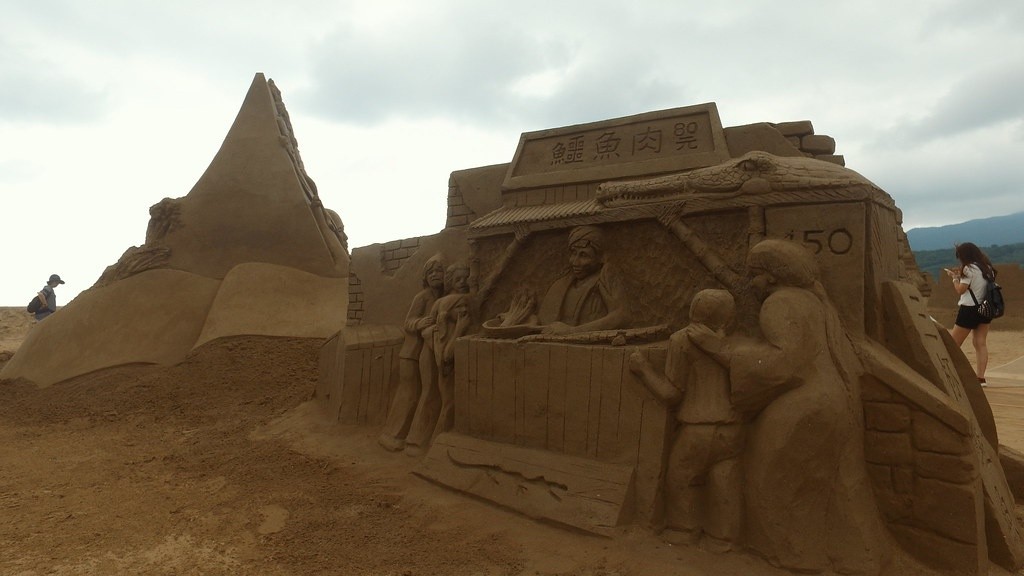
[27,295,42,313]
[967,262,1004,319]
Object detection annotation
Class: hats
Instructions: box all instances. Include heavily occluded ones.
[46,274,65,284]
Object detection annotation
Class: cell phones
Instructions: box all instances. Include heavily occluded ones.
[944,268,953,274]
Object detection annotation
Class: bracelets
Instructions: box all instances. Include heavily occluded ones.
[952,278,958,284]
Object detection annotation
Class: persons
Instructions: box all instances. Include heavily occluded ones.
[497,225,631,335]
[379,253,470,456]
[27,274,65,321]
[626,241,935,576]
[947,242,998,390]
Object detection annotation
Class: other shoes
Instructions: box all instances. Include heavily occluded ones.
[979,378,987,387]
